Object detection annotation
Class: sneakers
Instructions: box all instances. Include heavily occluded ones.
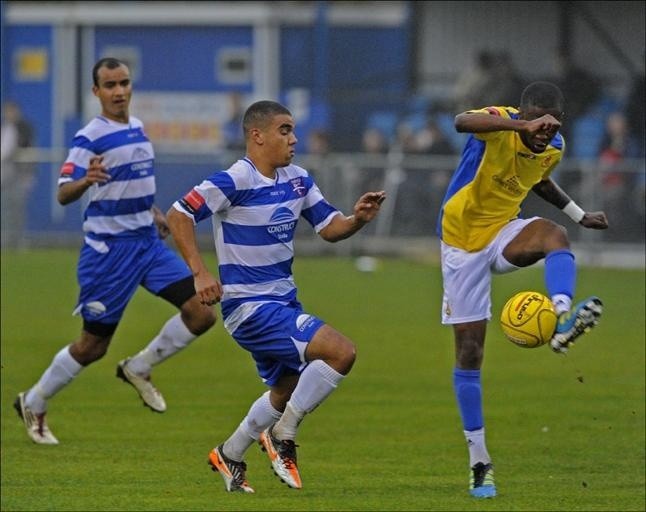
[116,356,169,414]
[12,388,60,448]
[468,458,496,500]
[548,295,605,354]
[259,423,303,489]
[206,444,255,494]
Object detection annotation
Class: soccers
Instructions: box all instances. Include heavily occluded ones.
[501,292,556,347]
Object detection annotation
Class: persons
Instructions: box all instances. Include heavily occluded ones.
[162,99,387,495]
[301,120,456,239]
[433,81,610,501]
[11,55,218,446]
[222,91,253,151]
[594,109,642,200]
[0,100,39,232]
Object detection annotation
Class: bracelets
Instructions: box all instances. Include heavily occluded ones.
[561,200,586,225]
